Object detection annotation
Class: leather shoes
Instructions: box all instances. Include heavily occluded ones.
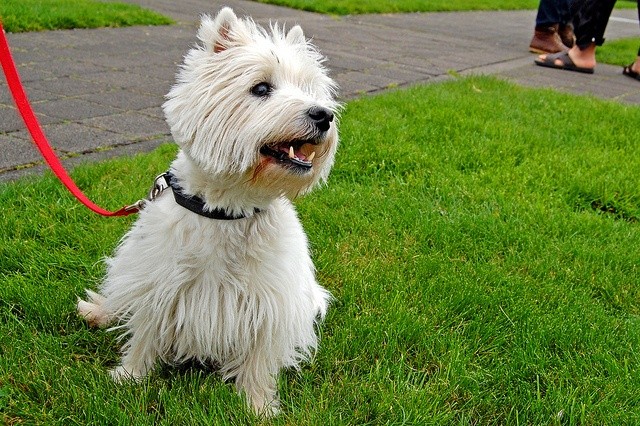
[559,21,576,47]
[529,26,571,55]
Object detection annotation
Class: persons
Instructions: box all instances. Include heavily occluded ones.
[530,0,576,53]
[534,0,639,79]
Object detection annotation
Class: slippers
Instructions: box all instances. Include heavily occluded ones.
[623,62,640,81]
[535,50,594,74]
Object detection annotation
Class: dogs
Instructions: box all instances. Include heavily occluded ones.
[76,6,338,418]
